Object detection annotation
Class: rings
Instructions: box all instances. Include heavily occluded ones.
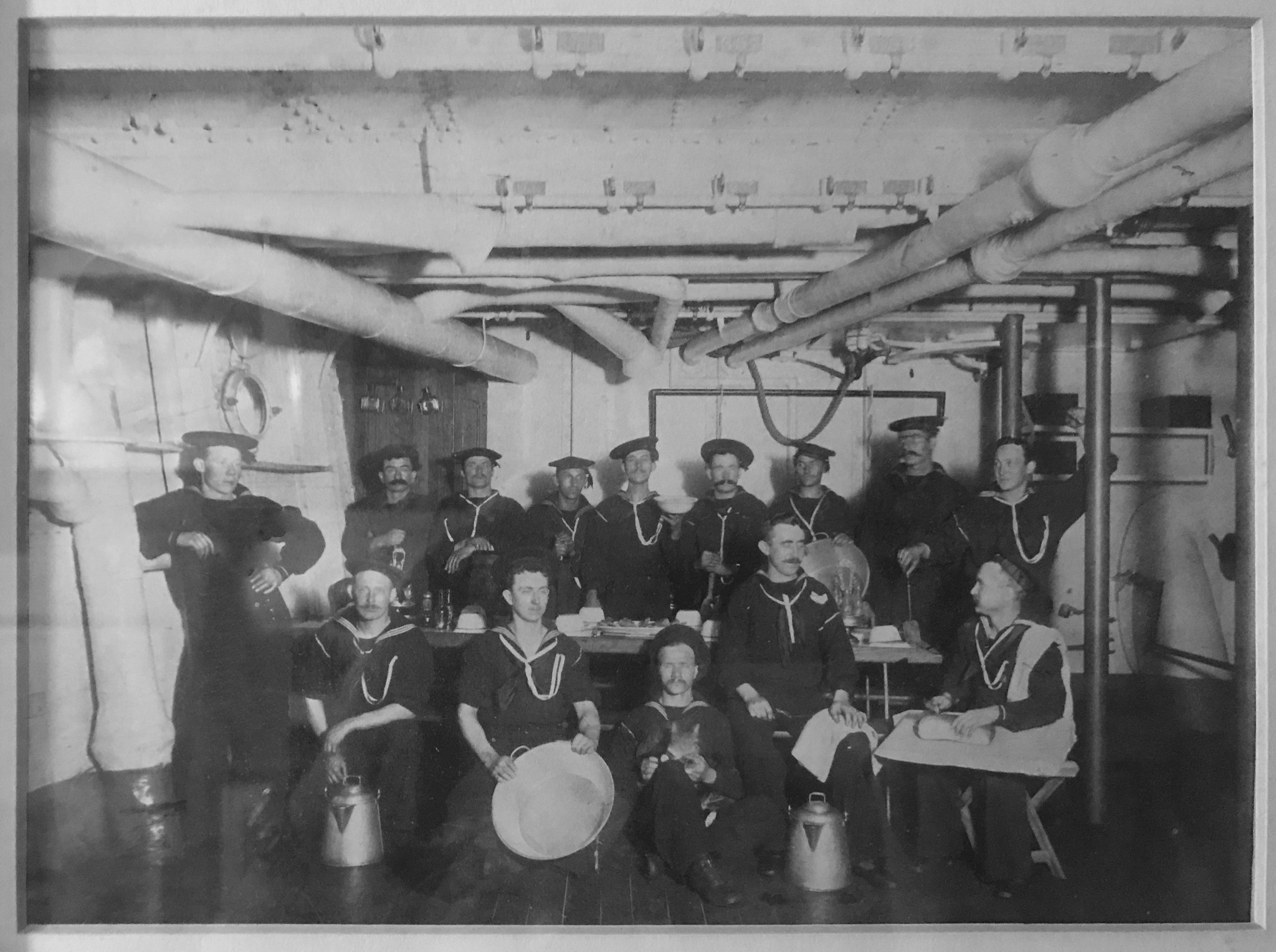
[474,544,480,548]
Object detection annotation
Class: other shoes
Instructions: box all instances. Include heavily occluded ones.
[689,857,745,909]
[753,854,777,875]
[644,852,666,878]
[853,862,898,889]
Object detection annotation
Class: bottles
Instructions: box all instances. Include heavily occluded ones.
[830,573,864,646]
[421,588,455,631]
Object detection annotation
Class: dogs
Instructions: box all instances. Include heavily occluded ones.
[662,717,736,828]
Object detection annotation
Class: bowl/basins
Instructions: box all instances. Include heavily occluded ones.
[554,606,723,642]
[868,625,902,643]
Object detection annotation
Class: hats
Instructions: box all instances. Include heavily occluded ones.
[455,447,504,465]
[181,430,258,452]
[699,439,754,467]
[889,415,946,434]
[548,456,595,472]
[610,435,657,459]
[650,621,712,684]
[795,444,837,462]
[494,546,560,585]
[343,555,409,590]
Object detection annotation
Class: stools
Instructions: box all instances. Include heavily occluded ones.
[1025,760,1077,880]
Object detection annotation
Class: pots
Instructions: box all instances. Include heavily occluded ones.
[800,532,870,610]
[490,740,615,862]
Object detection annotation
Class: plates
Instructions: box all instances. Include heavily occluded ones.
[596,621,666,638]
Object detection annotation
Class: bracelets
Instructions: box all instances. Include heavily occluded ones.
[992,704,1004,724]
[317,726,331,741]
[273,565,288,582]
[170,530,185,546]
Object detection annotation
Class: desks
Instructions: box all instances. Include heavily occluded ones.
[283,600,945,834]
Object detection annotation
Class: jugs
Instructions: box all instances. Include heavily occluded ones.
[785,792,854,893]
[320,774,385,867]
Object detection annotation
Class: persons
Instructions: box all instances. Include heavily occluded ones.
[860,415,968,651]
[341,443,438,605]
[708,513,884,879]
[610,624,787,908]
[279,558,435,883]
[527,457,612,620]
[584,438,683,622]
[134,432,326,861]
[680,438,768,619]
[441,557,602,877]
[426,446,527,627]
[913,549,1077,889]
[766,444,855,546]
[896,408,1118,627]
